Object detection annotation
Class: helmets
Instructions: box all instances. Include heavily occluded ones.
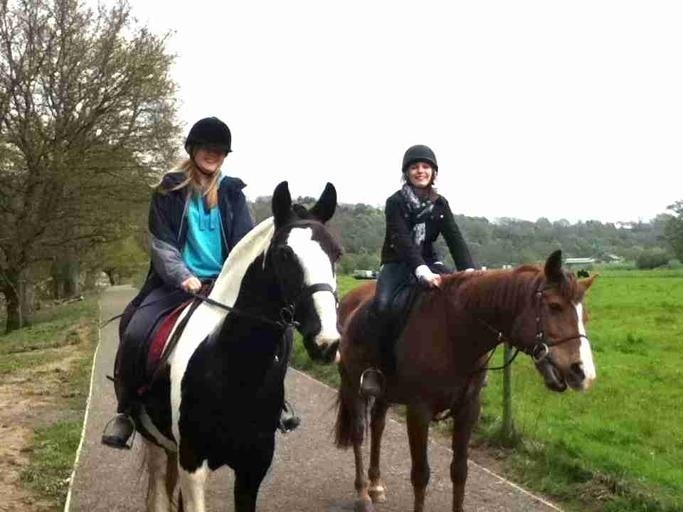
[187,116,232,154]
[402,144,438,171]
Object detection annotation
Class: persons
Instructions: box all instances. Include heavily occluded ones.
[97,115,303,451]
[354,143,477,399]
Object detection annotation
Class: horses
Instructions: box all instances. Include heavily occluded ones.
[325,245,598,512]
[116,178,344,512]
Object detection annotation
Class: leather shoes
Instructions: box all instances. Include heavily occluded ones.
[107,415,135,443]
[361,370,380,396]
[278,409,300,429]
